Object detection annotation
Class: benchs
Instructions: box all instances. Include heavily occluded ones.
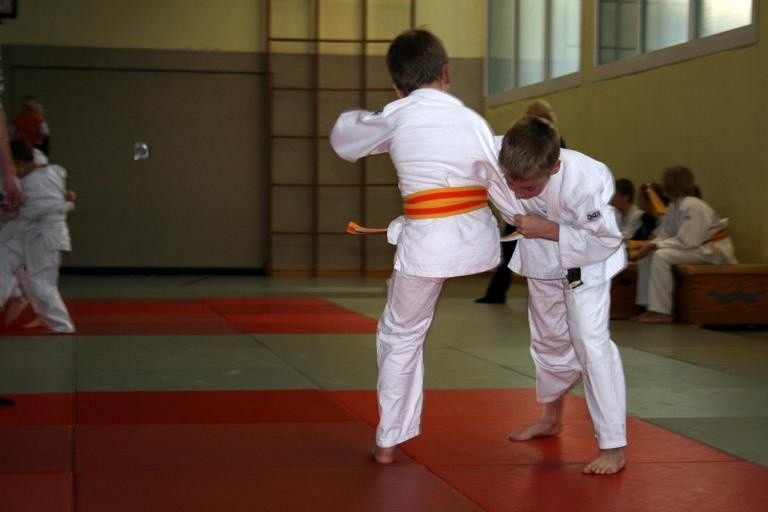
[609,260,767,326]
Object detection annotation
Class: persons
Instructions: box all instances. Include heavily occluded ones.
[473,165,734,324]
[493,100,629,474]
[1,59,76,336]
[331,28,528,463]
